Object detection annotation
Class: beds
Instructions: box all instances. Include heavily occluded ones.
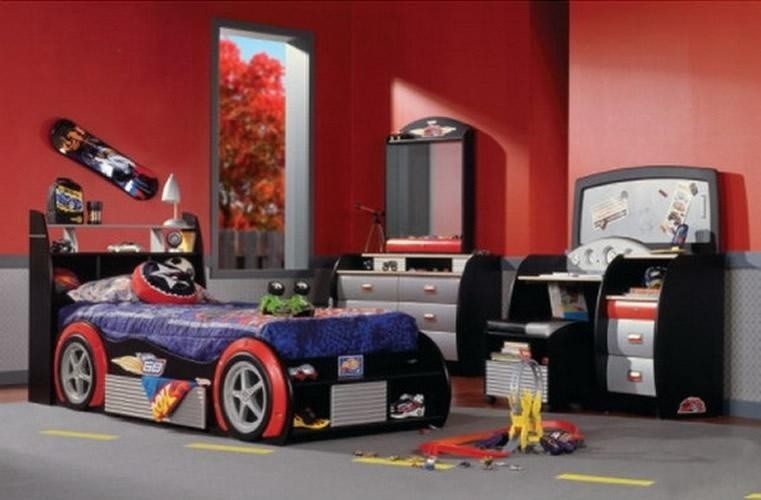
[25,207,453,446]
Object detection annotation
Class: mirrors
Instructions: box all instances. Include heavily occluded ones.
[380,116,474,254]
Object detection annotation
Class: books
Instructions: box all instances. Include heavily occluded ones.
[489,341,531,363]
[548,281,590,321]
[604,288,660,302]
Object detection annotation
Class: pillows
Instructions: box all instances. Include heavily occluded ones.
[64,256,210,308]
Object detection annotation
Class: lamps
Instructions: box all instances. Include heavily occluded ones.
[162,171,186,229]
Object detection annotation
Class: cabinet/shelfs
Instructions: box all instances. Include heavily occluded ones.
[480,253,598,408]
[596,249,724,419]
[329,251,501,374]
